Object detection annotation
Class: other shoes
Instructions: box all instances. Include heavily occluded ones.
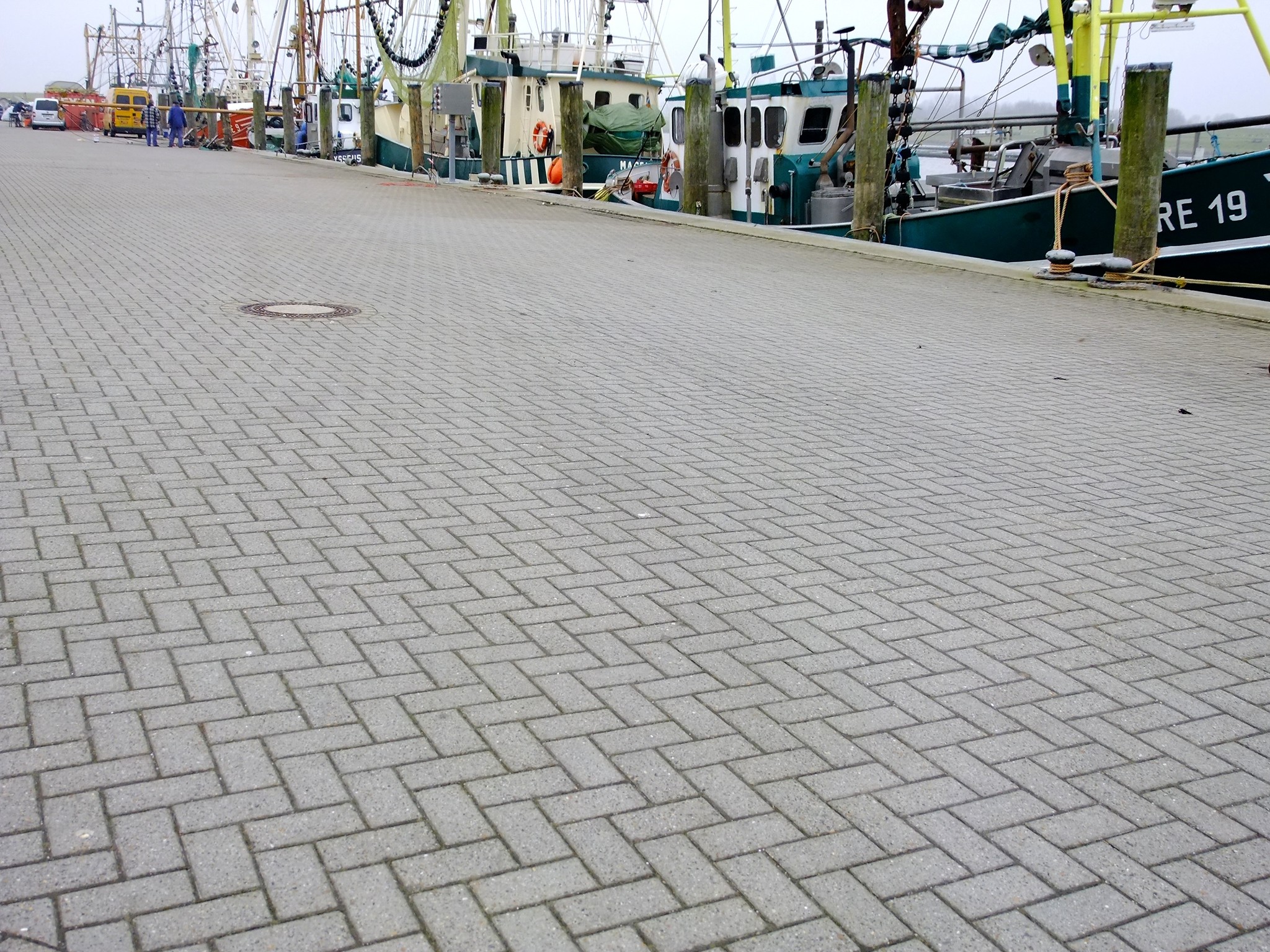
[179,146,182,148]
[168,145,172,147]
[148,145,151,146]
[154,145,158,146]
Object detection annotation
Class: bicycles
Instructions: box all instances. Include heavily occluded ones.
[79,113,93,132]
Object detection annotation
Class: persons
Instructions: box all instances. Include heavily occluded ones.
[79,110,89,131]
[167,100,188,148]
[140,100,161,147]
[12,102,27,128]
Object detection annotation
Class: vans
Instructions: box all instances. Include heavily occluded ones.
[97,87,153,140]
[30,97,67,131]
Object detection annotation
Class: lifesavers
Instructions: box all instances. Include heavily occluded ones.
[532,122,548,152]
[661,150,680,185]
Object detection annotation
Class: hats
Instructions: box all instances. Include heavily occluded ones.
[172,101,179,104]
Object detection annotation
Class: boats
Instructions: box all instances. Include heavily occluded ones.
[368,1,681,198]
[186,0,291,148]
[593,0,1270,300]
[246,0,390,165]
